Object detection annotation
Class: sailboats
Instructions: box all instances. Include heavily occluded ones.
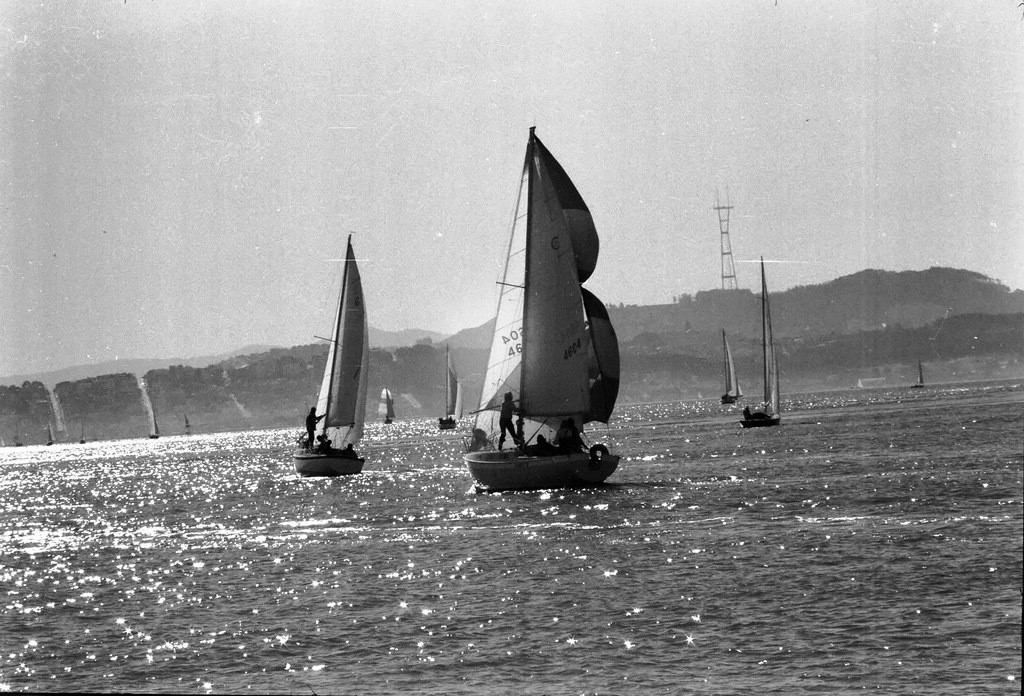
[293,233,369,478]
[78,424,85,444]
[184,414,191,434]
[849,379,863,390]
[46,425,52,446]
[909,360,925,388]
[461,126,621,493]
[739,256,781,428]
[719,329,742,404]
[377,388,396,424]
[436,344,461,430]
[148,409,160,439]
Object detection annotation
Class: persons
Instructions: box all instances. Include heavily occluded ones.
[316,435,333,454]
[340,444,358,459]
[534,418,588,456]
[743,406,753,421]
[498,391,520,451]
[306,407,323,447]
